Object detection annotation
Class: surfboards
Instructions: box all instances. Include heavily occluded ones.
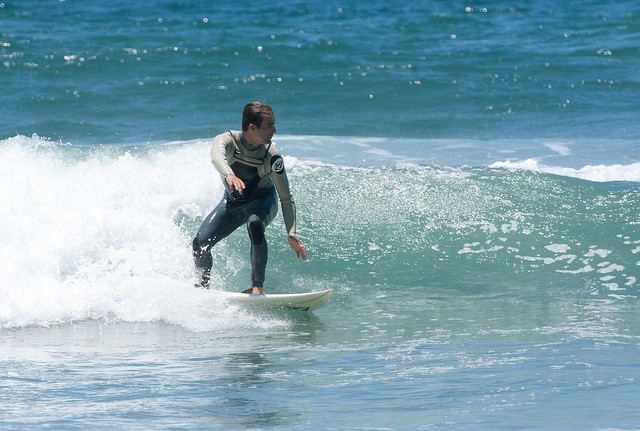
[149,267,333,313]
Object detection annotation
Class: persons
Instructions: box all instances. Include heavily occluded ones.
[193,103,307,295]
